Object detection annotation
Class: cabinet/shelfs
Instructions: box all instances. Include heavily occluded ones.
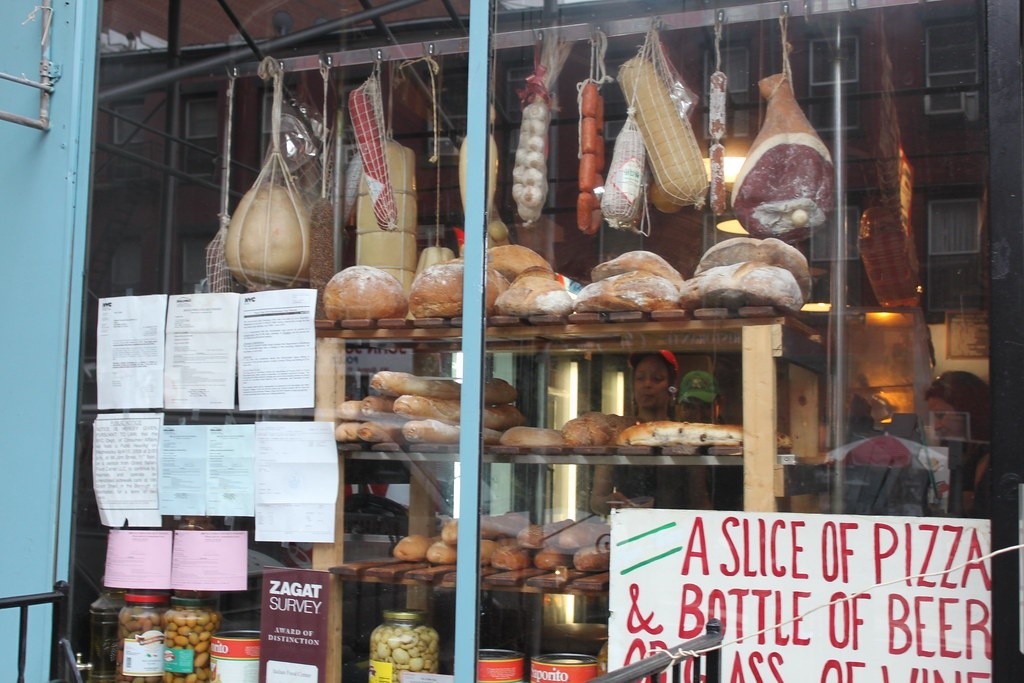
[312,310,838,683]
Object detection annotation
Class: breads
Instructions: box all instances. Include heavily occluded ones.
[391,510,610,573]
[334,369,791,450]
[322,239,811,316]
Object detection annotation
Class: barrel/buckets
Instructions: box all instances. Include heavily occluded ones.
[210,630,260,683]
[531,653,599,681]
[476,647,524,683]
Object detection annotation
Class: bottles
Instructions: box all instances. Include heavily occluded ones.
[368,607,439,683]
[89,577,221,683]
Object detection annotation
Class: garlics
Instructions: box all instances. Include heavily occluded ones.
[511,102,549,224]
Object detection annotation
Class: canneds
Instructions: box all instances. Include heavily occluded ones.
[209,629,260,683]
[115,594,222,683]
[368,607,440,683]
[477,647,599,683]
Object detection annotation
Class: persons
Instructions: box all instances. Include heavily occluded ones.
[925,371,992,508]
[679,371,723,423]
[589,350,712,513]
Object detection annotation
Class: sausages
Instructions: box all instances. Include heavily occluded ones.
[577,83,604,234]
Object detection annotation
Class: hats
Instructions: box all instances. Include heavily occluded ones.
[629,350,678,384]
[678,370,720,402]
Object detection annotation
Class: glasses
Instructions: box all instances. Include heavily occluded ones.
[932,374,948,393]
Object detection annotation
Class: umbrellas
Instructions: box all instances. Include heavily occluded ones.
[827,431,929,468]
